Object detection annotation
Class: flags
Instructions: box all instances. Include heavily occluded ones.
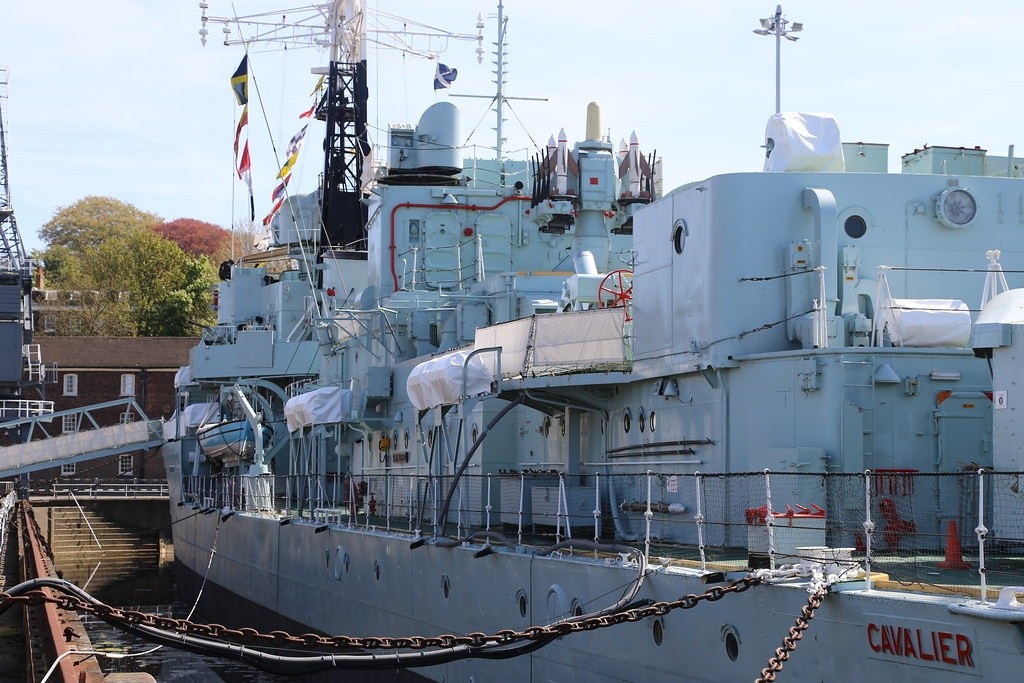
[434,63,458,91]
[263,73,325,226]
[230,54,255,221]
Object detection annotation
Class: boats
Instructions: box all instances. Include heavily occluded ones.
[163,1,1023,682]
